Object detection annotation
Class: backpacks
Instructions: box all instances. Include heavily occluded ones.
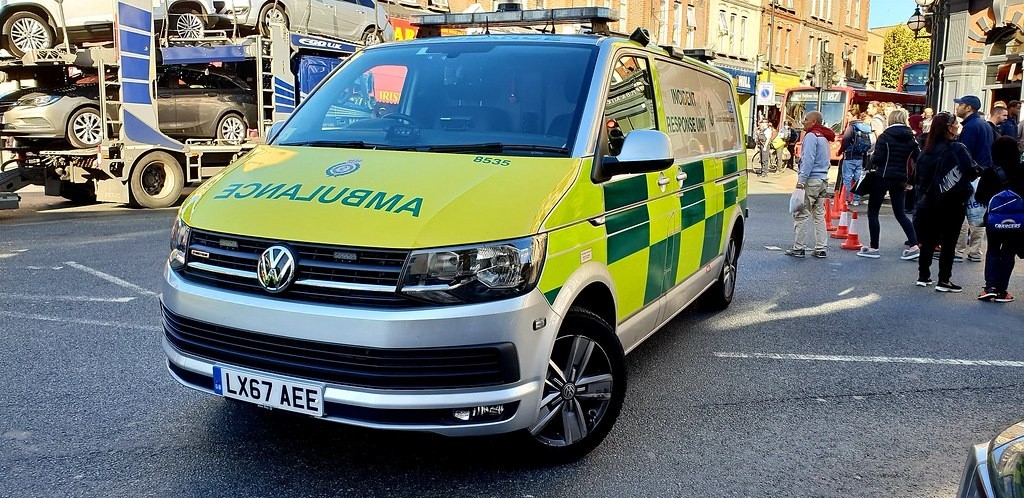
[845,122,871,153]
[987,165,1024,231]
[935,139,973,206]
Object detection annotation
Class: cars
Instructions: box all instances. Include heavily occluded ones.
[0,64,258,150]
[747,135,756,148]
[0,0,397,59]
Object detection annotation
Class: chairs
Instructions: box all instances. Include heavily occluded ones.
[544,69,583,135]
[432,58,508,131]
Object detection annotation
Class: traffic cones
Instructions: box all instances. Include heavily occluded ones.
[840,211,863,250]
[848,180,855,202]
[840,185,850,211]
[830,203,849,239]
[831,190,840,219]
[824,199,837,231]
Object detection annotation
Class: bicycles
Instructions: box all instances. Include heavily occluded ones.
[752,141,789,174]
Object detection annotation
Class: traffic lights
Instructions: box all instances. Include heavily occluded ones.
[806,64,819,87]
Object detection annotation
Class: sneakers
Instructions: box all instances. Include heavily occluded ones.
[995,289,1014,302]
[935,280,962,292]
[916,277,932,286]
[977,284,997,299]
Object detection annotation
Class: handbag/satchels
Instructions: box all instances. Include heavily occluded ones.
[850,159,880,195]
[789,184,805,214]
[782,147,791,161]
[965,175,987,227]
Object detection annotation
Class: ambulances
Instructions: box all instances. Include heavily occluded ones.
[158,3,749,464]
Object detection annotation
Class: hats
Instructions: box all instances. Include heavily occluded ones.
[953,95,981,110]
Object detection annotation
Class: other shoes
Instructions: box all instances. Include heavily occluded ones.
[812,249,826,257]
[850,200,859,206]
[901,249,920,259]
[968,253,981,261]
[857,246,880,258]
[953,256,963,262]
[785,248,805,257]
[932,250,941,260]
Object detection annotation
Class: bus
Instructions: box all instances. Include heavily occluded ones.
[897,61,930,95]
[780,87,927,160]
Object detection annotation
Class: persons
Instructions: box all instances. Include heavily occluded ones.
[974,135,1024,302]
[784,110,835,258]
[857,109,920,259]
[986,100,1024,162]
[952,95,993,262]
[755,118,799,178]
[836,100,902,206]
[914,110,979,293]
